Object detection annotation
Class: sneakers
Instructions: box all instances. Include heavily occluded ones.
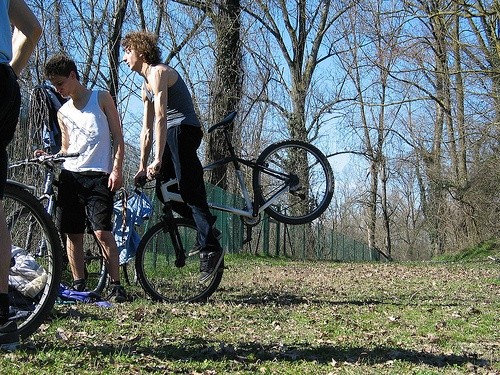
[0,321,20,351]
[188,227,223,256]
[64,284,85,303]
[111,286,127,305]
[198,247,225,284]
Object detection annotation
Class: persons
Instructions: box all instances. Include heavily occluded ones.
[0,0,43,349]
[42,55,124,303]
[122,32,225,282]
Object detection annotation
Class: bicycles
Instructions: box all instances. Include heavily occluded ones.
[65,182,153,294]
[7,152,110,297]
[1,178,63,344]
[134,112,335,304]
